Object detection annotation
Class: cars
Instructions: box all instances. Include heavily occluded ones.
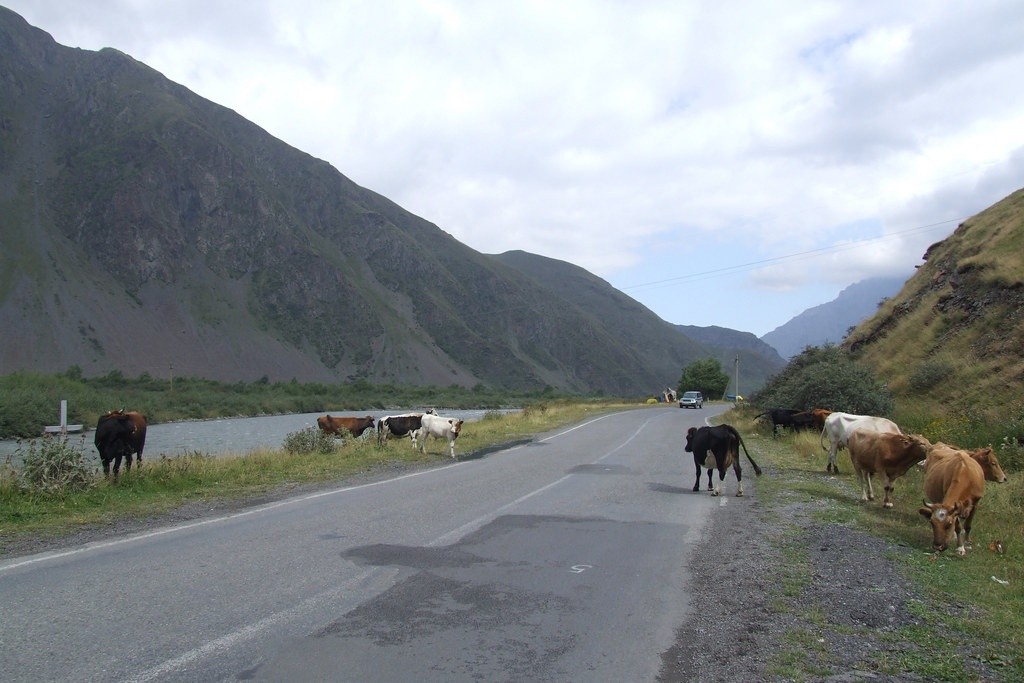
[679,392,704,409]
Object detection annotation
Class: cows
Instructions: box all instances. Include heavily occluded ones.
[317,415,375,439]
[685,424,762,497]
[378,406,464,459]
[94,406,146,481]
[753,407,1008,556]
